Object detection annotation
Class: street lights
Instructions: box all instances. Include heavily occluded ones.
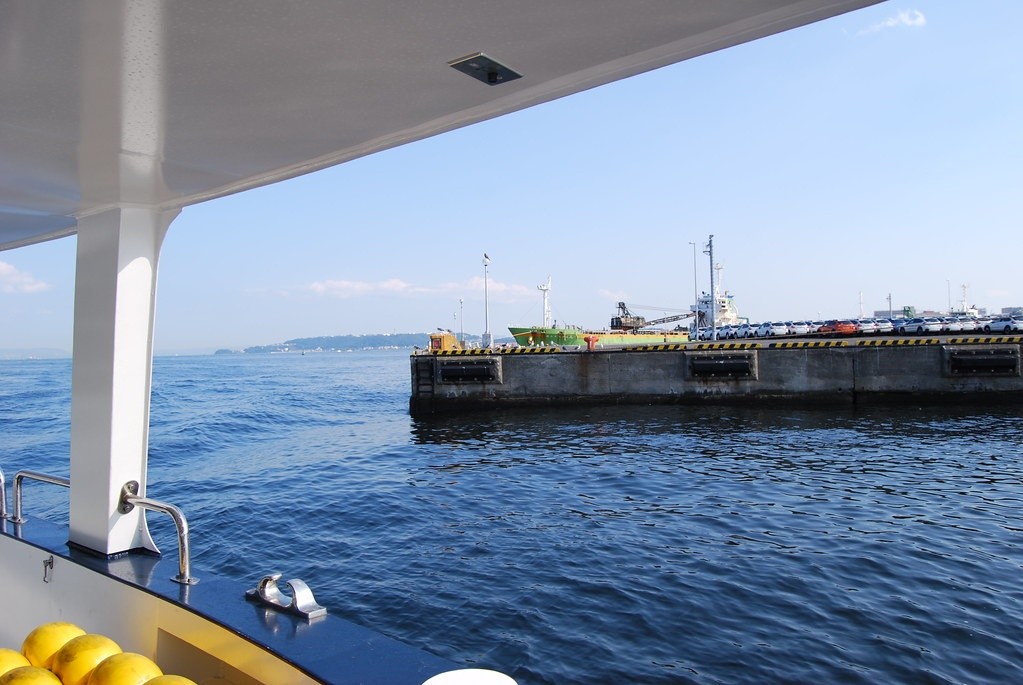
[482,257,491,334]
[459,298,465,341]
[454,313,457,338]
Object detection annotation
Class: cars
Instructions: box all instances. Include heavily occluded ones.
[688,309,1023,342]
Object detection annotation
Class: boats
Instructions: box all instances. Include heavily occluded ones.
[688,232,749,325]
[508,275,701,350]
